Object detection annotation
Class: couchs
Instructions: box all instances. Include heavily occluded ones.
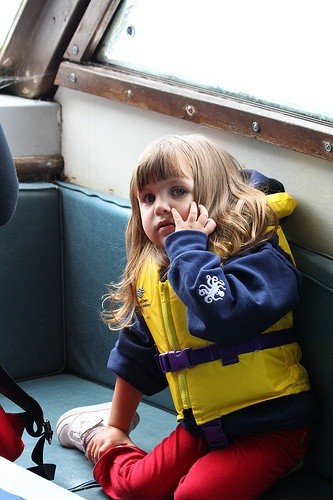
[0,176,333,500]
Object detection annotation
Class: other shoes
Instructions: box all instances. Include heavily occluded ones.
[56,401,141,452]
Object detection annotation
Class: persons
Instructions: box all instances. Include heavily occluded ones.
[50,131,313,500]
